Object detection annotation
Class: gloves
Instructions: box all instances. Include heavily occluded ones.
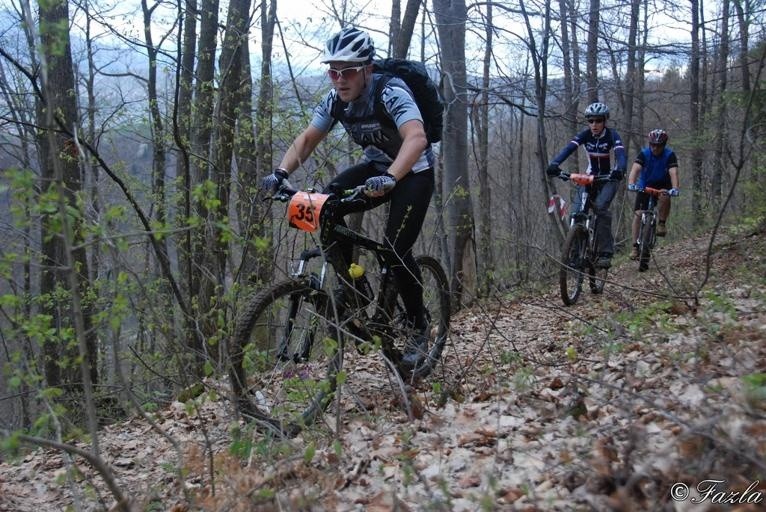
[628,183,639,192]
[609,170,625,181]
[546,164,562,176]
[668,188,679,197]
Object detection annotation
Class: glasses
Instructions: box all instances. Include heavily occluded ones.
[587,119,603,124]
[327,65,369,80]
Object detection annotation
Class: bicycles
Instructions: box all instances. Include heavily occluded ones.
[230,166,451,439]
[627,185,680,274]
[548,170,626,305]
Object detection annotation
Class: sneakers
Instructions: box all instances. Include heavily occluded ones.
[656,222,667,235]
[629,247,639,261]
[331,275,374,311]
[597,256,611,269]
[402,316,433,366]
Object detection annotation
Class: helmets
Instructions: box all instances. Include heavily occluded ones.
[320,27,375,64]
[584,103,609,121]
[648,129,668,145]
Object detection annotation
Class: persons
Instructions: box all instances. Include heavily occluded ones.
[260,28,435,366]
[546,102,627,268]
[627,129,679,260]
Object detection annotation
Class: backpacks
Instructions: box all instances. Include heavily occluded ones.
[327,58,443,143]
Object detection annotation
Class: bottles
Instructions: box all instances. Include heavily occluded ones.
[348,262,373,304]
